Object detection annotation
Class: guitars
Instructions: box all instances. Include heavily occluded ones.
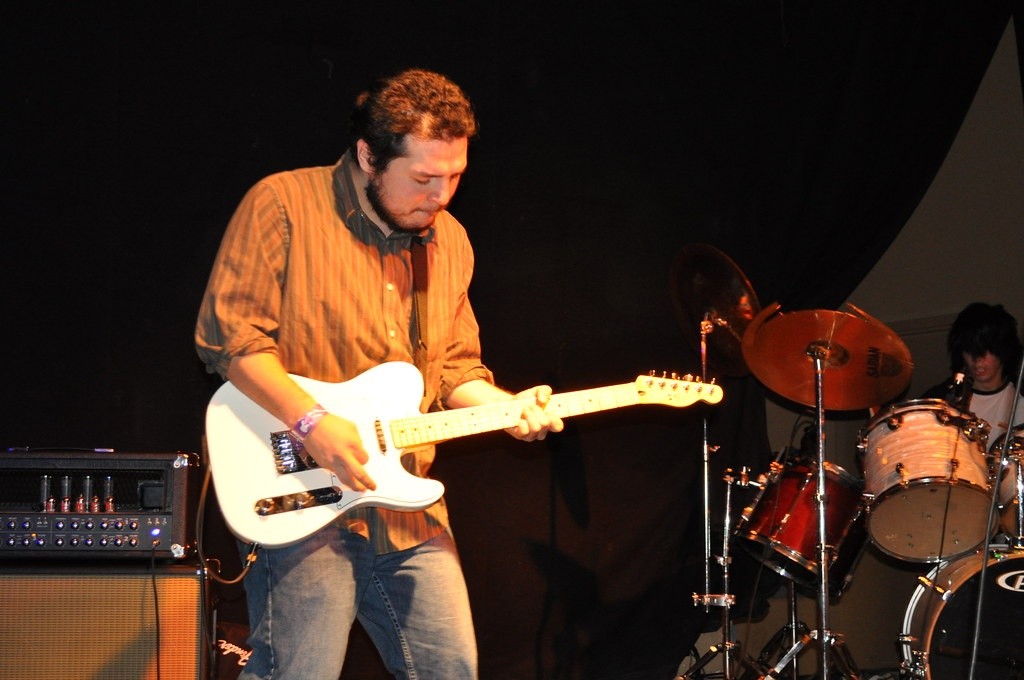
[202,361,723,550]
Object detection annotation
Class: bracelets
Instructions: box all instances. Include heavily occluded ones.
[290,403,327,443]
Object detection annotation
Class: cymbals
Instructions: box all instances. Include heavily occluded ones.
[666,241,764,381]
[738,300,915,412]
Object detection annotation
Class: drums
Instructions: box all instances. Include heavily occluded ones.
[860,395,1000,565]
[988,422,1024,540]
[732,452,871,605]
[901,546,1024,680]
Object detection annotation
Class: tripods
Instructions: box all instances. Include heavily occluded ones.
[676,311,865,680]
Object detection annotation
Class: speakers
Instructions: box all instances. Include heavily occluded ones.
[1,559,220,680]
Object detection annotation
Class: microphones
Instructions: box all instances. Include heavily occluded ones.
[946,368,968,401]
[801,424,818,453]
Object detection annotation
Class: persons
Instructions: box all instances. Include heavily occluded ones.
[196,70,564,680]
[921,303,1024,451]
